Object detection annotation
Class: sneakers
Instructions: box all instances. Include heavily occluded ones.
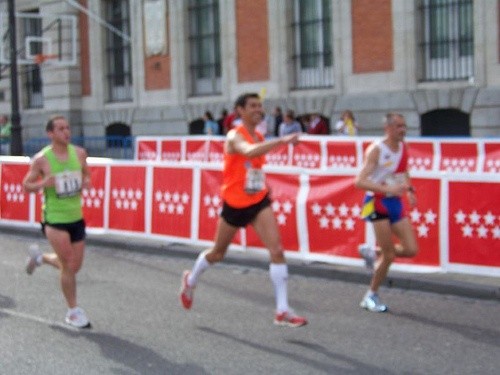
[273,310,307,328]
[358,244,375,277]
[360,295,387,312]
[65,308,91,328]
[25,245,41,275]
[180,272,194,310]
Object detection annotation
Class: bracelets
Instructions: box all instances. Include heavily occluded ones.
[407,188,414,192]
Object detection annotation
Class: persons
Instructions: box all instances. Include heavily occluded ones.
[205,107,358,139]
[181,93,309,328]
[355,112,419,311]
[25,115,93,327]
[0,114,11,155]
